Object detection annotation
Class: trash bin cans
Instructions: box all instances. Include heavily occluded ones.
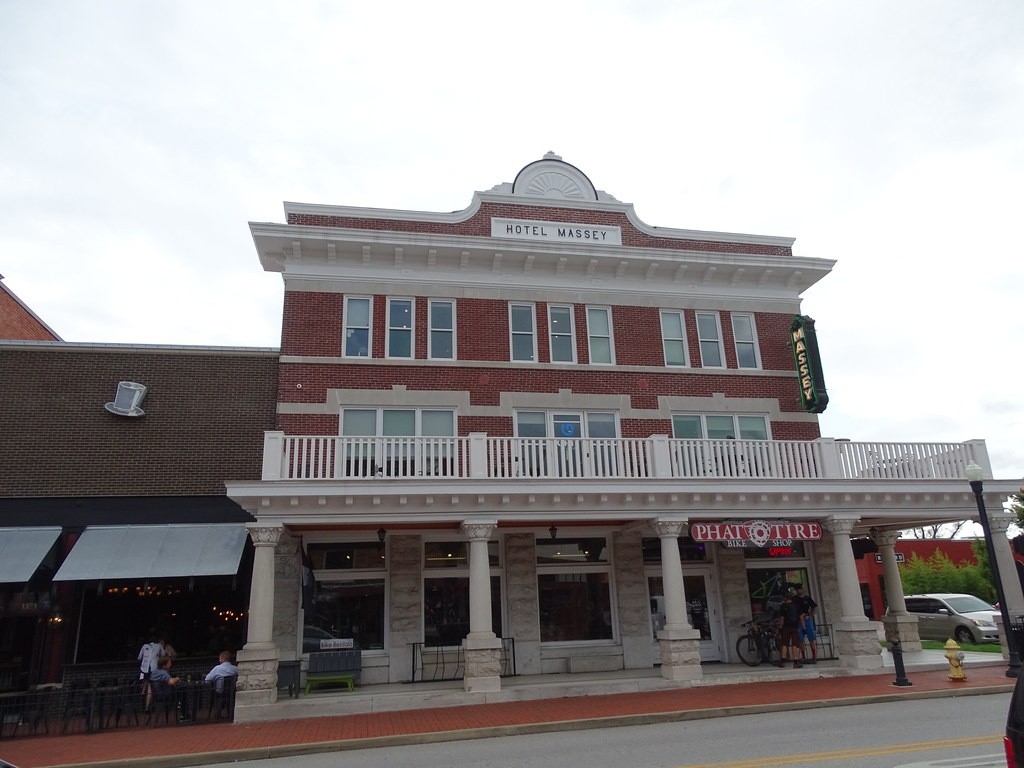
[1012,615,1024,663]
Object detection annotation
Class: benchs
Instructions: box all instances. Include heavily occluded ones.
[307,651,362,690]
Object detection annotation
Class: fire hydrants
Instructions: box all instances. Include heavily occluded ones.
[944,637,967,682]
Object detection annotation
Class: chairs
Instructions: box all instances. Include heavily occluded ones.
[0,675,237,740]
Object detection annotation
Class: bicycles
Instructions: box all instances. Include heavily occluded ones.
[736,616,790,668]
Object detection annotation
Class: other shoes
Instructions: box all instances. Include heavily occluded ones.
[145,707,153,712]
[180,714,191,723]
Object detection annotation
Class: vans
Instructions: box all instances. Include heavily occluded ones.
[885,593,1002,644]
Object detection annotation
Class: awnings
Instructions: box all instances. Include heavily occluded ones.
[0,525,249,583]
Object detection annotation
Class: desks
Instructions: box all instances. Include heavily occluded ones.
[277,660,303,699]
[304,674,357,694]
[174,682,213,726]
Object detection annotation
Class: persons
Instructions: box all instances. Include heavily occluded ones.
[205,650,238,693]
[772,592,803,668]
[151,655,191,723]
[791,583,817,664]
[137,637,177,710]
[692,599,705,638]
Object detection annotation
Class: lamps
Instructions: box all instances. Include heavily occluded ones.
[376,527,387,544]
[869,526,879,539]
[549,525,558,541]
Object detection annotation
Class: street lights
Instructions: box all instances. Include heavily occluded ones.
[964,459,1024,680]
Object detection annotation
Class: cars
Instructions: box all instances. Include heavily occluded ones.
[766,595,784,615]
[304,626,359,652]
[651,595,709,635]
